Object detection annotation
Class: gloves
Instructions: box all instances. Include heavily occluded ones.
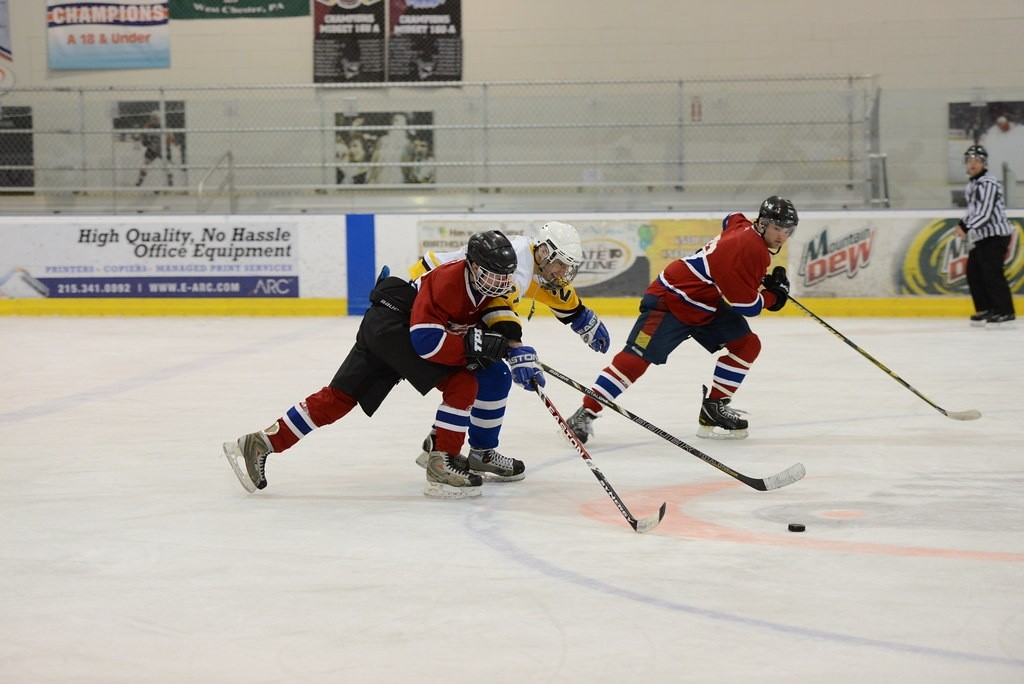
[766,267,791,312]
[572,310,610,354]
[510,346,544,392]
[466,329,508,365]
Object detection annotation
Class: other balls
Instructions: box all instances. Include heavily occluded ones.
[788,523,805,532]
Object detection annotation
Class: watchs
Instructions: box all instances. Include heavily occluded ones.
[960,219,971,228]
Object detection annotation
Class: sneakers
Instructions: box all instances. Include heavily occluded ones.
[698,386,748,438]
[426,452,483,498]
[225,431,271,493]
[988,312,1016,324]
[418,430,470,473]
[566,408,596,446]
[468,448,526,482]
[970,311,988,321]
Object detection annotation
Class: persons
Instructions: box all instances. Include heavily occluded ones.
[956,144,1017,323]
[116,119,186,195]
[567,196,799,447]
[336,113,434,183]
[222,220,610,500]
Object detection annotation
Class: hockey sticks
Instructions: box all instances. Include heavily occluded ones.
[531,377,670,533]
[787,294,983,421]
[536,360,808,492]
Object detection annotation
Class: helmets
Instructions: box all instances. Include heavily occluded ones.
[756,196,799,228]
[965,145,989,165]
[466,230,518,299]
[533,223,586,292]
